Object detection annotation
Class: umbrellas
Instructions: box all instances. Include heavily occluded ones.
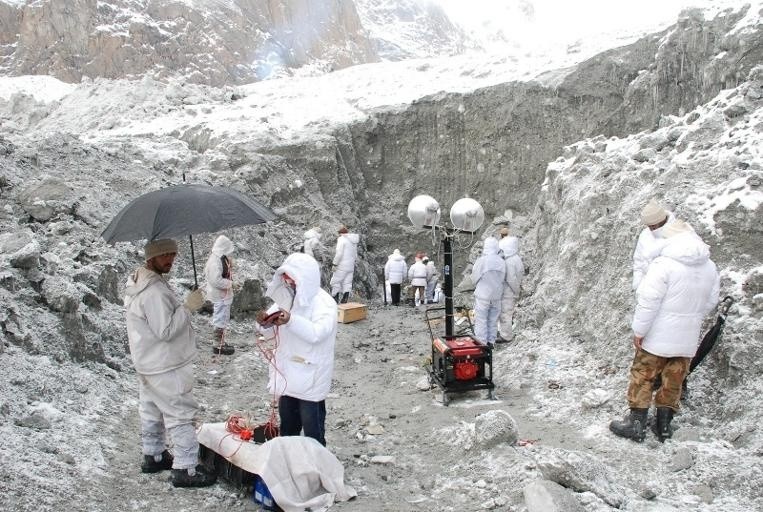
[98,168,280,301]
[678,294,734,383]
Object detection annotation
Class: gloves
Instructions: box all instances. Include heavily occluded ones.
[232,280,244,289]
[182,285,204,313]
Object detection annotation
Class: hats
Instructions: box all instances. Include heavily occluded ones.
[144,239,178,262]
[641,203,667,226]
[338,228,348,232]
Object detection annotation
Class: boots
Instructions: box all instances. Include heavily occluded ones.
[657,406,673,442]
[333,292,349,304]
[609,409,647,442]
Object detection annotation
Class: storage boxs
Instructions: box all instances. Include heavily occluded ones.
[337,302,369,324]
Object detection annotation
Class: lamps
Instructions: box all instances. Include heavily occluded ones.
[449,198,485,233]
[407,195,441,228]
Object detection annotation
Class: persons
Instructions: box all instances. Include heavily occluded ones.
[330,227,359,303]
[468,236,506,348]
[422,257,439,303]
[382,249,410,304]
[122,239,217,488]
[611,228,721,443]
[408,252,429,306]
[202,235,236,352]
[624,200,703,293]
[501,235,524,346]
[261,252,338,445]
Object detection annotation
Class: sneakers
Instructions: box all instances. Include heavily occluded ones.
[495,331,512,343]
[141,449,174,473]
[172,469,217,487]
[213,342,234,355]
